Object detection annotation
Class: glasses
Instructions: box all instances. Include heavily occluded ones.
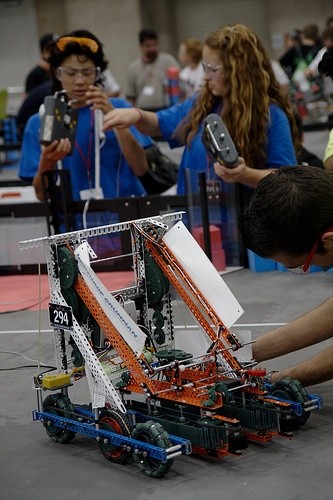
[202,60,224,78]
[285,225,333,275]
[56,67,101,83]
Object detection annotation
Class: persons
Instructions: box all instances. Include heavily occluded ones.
[240,165,333,388]
[16,24,333,264]
